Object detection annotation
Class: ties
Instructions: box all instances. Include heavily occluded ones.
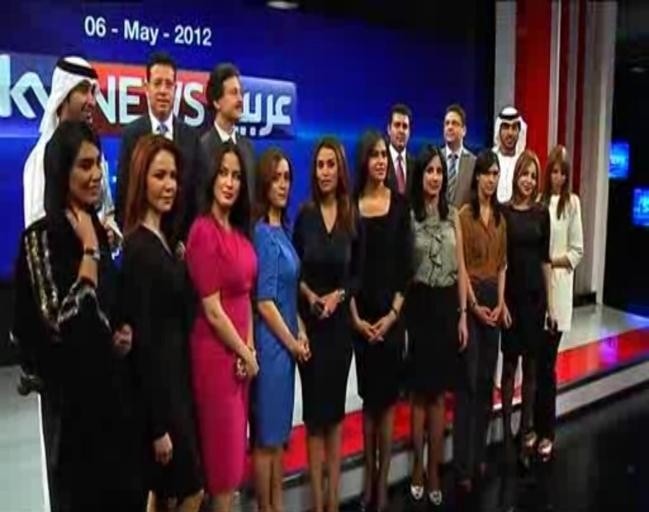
[396,155,405,196]
[159,123,167,136]
[447,152,457,203]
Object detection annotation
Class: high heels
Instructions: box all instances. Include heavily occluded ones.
[520,430,555,467]
[408,467,425,501]
[425,473,445,507]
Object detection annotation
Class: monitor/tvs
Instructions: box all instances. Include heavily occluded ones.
[629,186,649,230]
[608,137,634,182]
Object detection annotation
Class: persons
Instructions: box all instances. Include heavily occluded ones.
[500,147,556,475]
[250,143,312,512]
[349,129,414,512]
[522,146,584,460]
[290,135,354,512]
[13,120,142,512]
[186,140,258,512]
[120,135,207,512]
